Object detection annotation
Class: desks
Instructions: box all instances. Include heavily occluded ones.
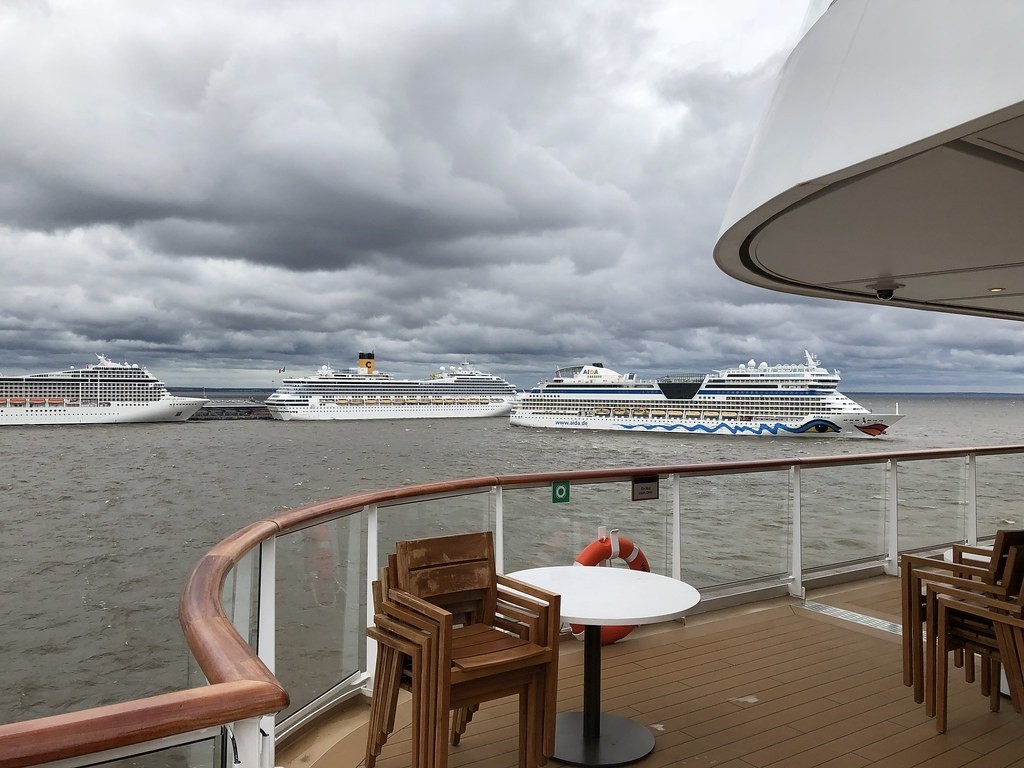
[496,566,701,768]
[944,544,1024,697]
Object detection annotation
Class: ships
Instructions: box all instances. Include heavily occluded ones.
[263,351,518,421]
[0,352,210,426]
[507,350,905,438]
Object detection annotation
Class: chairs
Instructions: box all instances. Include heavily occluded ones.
[365,531,561,768]
[900,529,1024,734]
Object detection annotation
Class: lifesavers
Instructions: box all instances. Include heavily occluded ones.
[569,537,650,645]
